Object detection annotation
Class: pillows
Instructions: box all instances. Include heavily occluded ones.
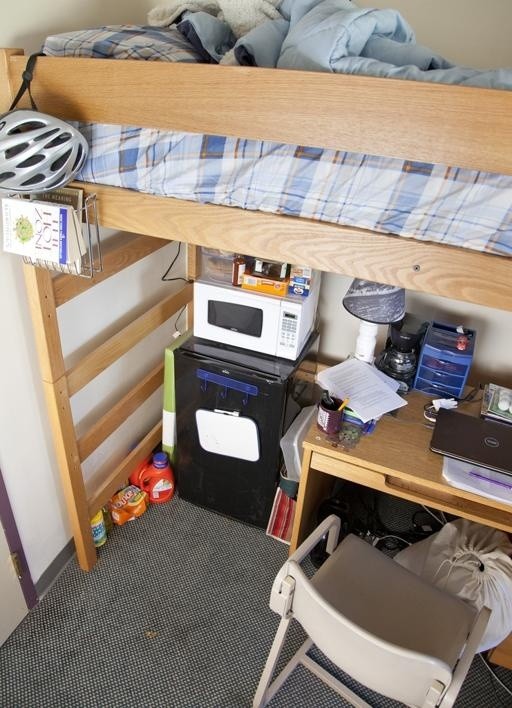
[41,24,208,64]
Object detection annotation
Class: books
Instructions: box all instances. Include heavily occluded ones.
[314,353,408,435]
[2,186,89,275]
[480,383,512,427]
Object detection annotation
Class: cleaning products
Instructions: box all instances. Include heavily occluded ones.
[127,445,154,487]
[140,452,174,503]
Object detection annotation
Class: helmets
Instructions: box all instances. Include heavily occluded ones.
[0,110,89,196]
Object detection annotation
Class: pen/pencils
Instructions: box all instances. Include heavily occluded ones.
[319,390,350,410]
[362,418,382,435]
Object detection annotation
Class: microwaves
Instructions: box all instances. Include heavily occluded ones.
[193,275,321,360]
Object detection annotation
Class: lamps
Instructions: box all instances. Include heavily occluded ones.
[341,278,406,365]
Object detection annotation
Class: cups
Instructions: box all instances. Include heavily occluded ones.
[316,398,344,435]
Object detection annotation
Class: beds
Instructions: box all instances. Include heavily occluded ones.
[1,45,512,262]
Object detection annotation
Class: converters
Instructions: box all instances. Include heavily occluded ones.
[415,525,443,538]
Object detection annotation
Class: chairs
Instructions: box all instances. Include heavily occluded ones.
[251,513,492,708]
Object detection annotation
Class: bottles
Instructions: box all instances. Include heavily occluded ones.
[232,255,246,286]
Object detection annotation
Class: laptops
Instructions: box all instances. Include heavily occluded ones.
[430,407,512,477]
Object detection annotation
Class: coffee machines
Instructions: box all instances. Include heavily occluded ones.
[377,312,430,393]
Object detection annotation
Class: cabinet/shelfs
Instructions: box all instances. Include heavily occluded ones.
[413,322,476,400]
[173,331,320,531]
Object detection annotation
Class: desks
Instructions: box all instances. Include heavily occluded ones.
[287,384,511,559]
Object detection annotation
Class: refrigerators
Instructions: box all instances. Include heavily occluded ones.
[174,335,296,531]
